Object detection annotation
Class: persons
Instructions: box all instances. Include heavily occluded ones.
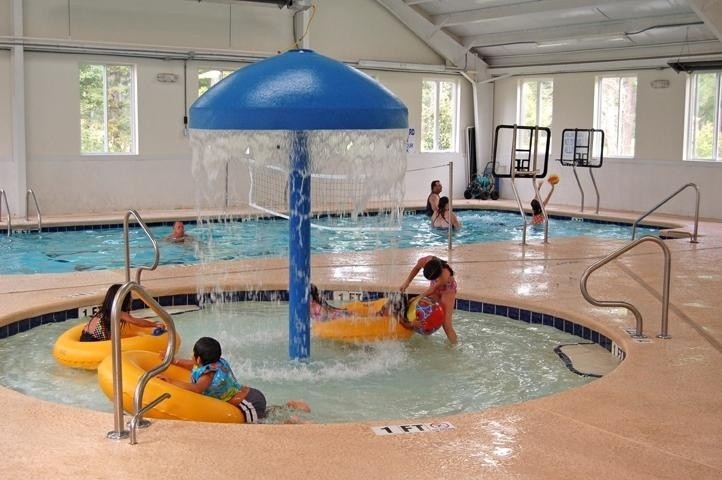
[79,283,165,342]
[396,256,460,346]
[306,283,409,322]
[428,195,460,231]
[425,180,442,216]
[530,180,554,226]
[156,335,312,424]
[159,222,196,244]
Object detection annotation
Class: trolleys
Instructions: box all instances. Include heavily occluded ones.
[464,161,499,201]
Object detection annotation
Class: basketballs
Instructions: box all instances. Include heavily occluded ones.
[548,174,559,184]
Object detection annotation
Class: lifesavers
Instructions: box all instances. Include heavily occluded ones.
[314,299,413,343]
[52,319,181,370]
[97,350,245,423]
